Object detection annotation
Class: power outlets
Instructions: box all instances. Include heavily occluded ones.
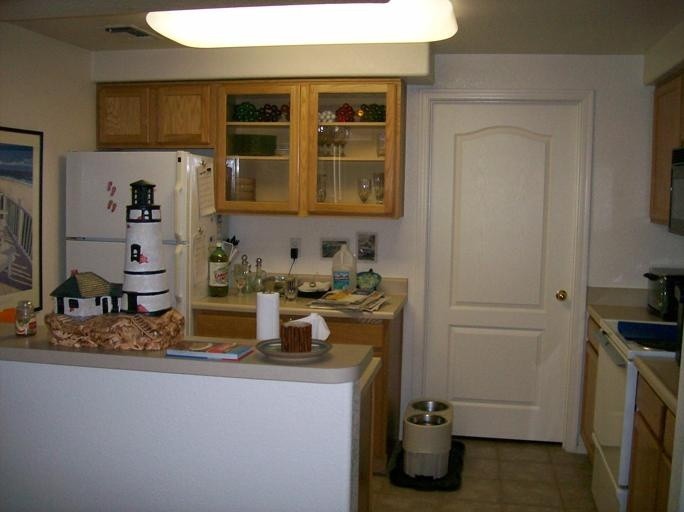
[286,236,303,263]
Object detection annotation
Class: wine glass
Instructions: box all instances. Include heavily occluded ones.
[358,176,371,204]
[234,264,247,297]
[318,126,350,157]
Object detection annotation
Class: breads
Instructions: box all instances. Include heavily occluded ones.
[280,322,311,352]
[45,309,185,351]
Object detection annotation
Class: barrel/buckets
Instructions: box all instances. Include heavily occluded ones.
[331,244,357,291]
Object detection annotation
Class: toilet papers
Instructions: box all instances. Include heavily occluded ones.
[256,293,280,343]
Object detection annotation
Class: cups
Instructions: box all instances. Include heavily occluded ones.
[377,134,385,159]
[317,175,326,202]
[285,277,298,301]
[373,173,383,204]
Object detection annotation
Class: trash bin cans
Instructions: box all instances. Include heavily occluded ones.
[402,396,454,480]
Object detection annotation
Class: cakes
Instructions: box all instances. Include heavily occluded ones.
[298,281,331,300]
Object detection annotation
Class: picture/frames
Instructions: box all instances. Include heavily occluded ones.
[0,125,44,312]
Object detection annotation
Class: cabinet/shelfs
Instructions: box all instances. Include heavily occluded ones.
[95,77,407,219]
[645,57,684,225]
[0,323,382,512]
[581,303,683,512]
[192,272,408,475]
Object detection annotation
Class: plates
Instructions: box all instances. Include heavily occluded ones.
[357,271,381,289]
[256,339,331,358]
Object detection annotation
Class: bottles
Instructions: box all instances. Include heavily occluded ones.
[209,244,229,297]
[240,254,266,292]
[15,300,37,337]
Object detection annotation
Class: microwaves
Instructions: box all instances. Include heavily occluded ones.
[669,149,684,236]
[643,268,684,322]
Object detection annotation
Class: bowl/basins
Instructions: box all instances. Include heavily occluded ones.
[229,134,276,156]
[229,177,256,200]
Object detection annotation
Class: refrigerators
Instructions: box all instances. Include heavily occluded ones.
[65,150,218,339]
